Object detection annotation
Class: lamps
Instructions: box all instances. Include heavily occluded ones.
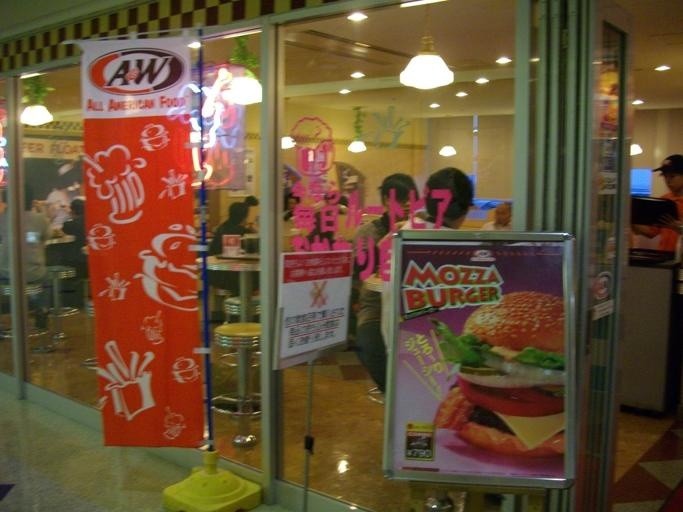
[20,105,53,126]
[221,67,262,105]
[400,4,455,90]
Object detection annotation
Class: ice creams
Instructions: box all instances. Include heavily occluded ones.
[87,147,143,224]
[142,310,165,345]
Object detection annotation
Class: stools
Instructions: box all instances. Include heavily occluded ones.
[218,296,274,388]
[44,266,77,319]
[210,322,262,446]
[0,282,49,339]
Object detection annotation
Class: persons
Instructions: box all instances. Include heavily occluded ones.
[0,180,51,329]
[204,201,260,292]
[631,154,682,414]
[52,198,88,272]
[244,195,260,234]
[350,173,420,392]
[281,193,297,219]
[374,168,474,350]
[480,200,511,230]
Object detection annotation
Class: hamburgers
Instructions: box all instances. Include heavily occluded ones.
[434,291,566,458]
[88,225,115,250]
[140,233,199,312]
[173,358,199,383]
[141,123,168,151]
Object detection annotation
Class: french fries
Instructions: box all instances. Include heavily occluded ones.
[159,169,188,198]
[98,273,130,296]
[97,341,154,385]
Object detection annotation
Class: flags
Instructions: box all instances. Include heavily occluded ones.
[73,39,206,452]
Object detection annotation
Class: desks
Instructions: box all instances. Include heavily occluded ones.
[205,255,261,419]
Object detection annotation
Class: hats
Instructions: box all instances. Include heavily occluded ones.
[650,155,683,174]
